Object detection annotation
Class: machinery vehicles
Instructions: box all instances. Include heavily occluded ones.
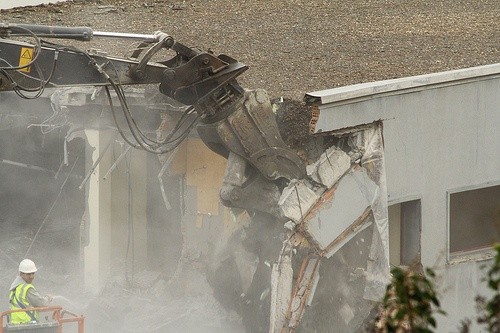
[1,23,395,333]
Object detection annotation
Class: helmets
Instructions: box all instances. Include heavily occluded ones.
[19,259,37,273]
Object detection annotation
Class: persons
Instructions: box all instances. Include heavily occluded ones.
[7,259,54,324]
